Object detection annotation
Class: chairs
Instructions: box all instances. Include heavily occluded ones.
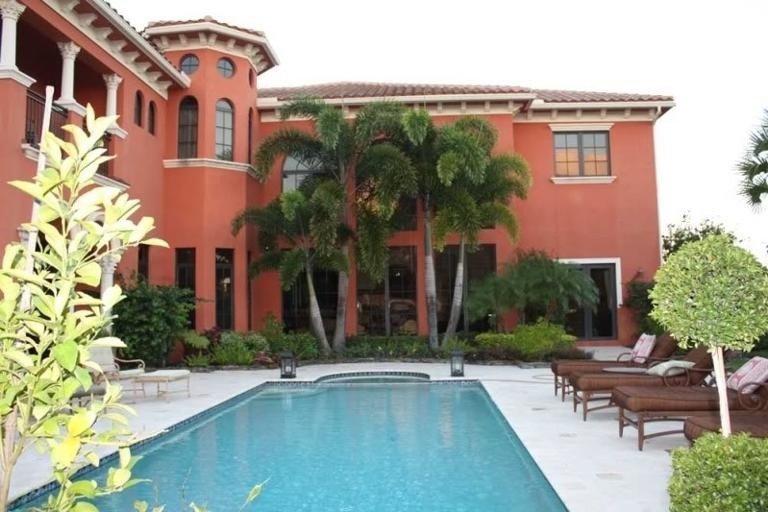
[67,346,146,417]
[550,330,768,454]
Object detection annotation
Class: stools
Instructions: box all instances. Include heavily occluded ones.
[138,368,191,401]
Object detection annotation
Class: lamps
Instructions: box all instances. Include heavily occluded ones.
[279,351,297,377]
[450,346,465,376]
